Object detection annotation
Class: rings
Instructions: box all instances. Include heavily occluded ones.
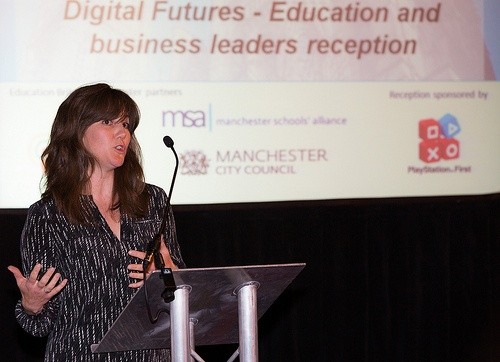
[45,287,51,293]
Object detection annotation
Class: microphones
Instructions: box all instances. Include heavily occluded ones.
[153,135,179,239]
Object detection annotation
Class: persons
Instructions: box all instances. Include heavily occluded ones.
[6,84,189,362]
